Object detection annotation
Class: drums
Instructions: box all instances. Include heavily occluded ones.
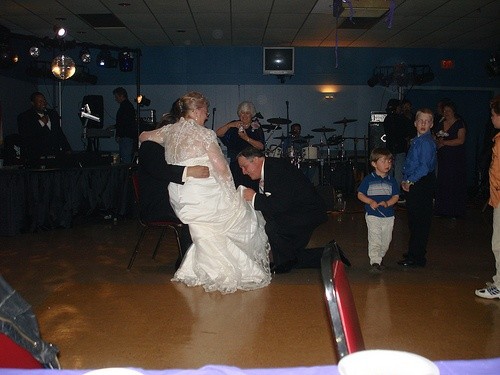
[268,144,282,158]
[302,146,318,159]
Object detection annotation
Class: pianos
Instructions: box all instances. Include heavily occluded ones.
[81,128,114,153]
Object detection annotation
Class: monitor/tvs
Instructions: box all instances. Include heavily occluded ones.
[264,46,294,75]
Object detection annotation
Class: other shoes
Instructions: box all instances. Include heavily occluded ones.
[369,257,383,273]
[329,240,351,266]
[270,262,288,273]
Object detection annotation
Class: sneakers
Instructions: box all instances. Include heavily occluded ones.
[475,282,500,299]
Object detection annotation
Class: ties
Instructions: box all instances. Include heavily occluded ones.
[259,178,265,194]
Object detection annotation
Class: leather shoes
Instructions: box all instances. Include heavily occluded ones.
[397,253,422,267]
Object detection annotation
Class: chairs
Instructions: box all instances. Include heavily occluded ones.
[320,240,365,360]
[0,276,43,370]
[127,176,183,271]
[4,134,23,166]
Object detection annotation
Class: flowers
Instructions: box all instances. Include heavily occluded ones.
[251,121,260,129]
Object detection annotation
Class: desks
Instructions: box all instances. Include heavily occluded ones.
[0,166,127,236]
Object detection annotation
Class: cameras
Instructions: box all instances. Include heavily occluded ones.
[238,124,244,131]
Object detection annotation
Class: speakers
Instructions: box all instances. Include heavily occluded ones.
[82,95,104,127]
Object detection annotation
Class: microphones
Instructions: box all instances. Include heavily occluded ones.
[44,109,48,126]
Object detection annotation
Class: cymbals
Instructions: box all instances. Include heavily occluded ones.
[290,138,307,142]
[312,128,336,132]
[262,125,281,129]
[303,136,314,138]
[267,118,292,124]
[333,119,357,124]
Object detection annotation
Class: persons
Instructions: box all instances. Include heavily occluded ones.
[20,91,72,159]
[216,101,267,190]
[137,93,276,293]
[400,101,414,120]
[399,108,438,266]
[382,99,411,205]
[359,146,400,269]
[435,103,466,220]
[132,112,209,274]
[475,97,500,298]
[280,124,301,153]
[113,88,139,163]
[236,148,351,272]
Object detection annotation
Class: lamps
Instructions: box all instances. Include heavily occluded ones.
[368,62,434,88]
[321,92,339,99]
[0,20,151,135]
